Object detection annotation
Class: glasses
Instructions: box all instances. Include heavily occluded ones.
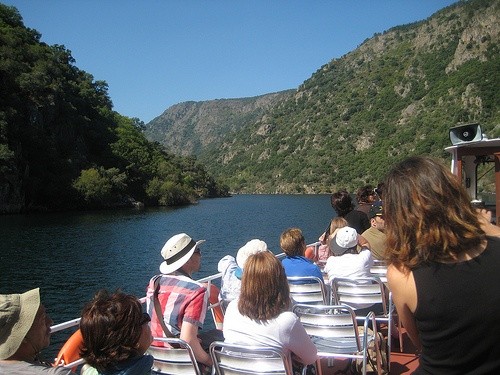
[141,313,151,326]
[194,248,201,256]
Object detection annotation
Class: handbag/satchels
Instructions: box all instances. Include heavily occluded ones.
[292,359,316,375]
[197,329,224,352]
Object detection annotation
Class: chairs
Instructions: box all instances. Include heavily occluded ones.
[63,259,405,375]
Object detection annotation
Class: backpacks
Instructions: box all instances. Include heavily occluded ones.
[352,325,389,375]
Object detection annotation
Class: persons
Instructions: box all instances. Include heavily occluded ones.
[384,156,500,375]
[0,183,387,375]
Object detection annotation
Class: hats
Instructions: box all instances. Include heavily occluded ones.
[369,202,385,219]
[236,239,273,270]
[330,227,358,255]
[159,232,206,275]
[0,287,41,360]
